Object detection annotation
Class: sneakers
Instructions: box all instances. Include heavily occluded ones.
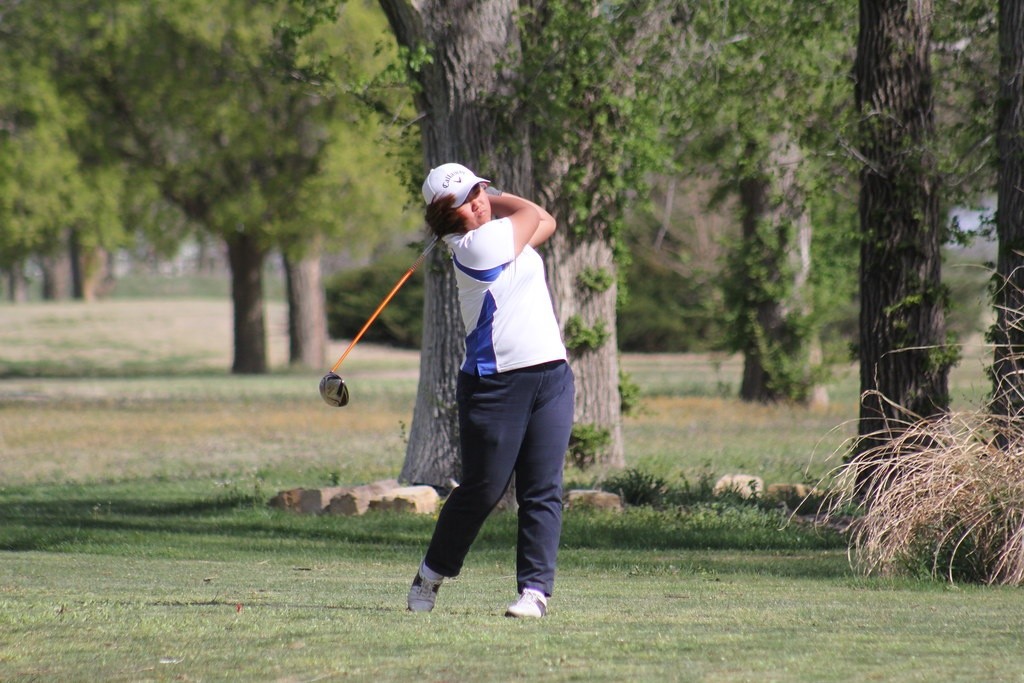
[505,587,546,616]
[408,560,444,612]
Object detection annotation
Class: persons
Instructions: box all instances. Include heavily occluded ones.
[408,162,575,618]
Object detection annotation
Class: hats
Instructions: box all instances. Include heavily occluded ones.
[423,161,491,209]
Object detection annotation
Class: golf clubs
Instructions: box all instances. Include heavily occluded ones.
[319,234,441,408]
